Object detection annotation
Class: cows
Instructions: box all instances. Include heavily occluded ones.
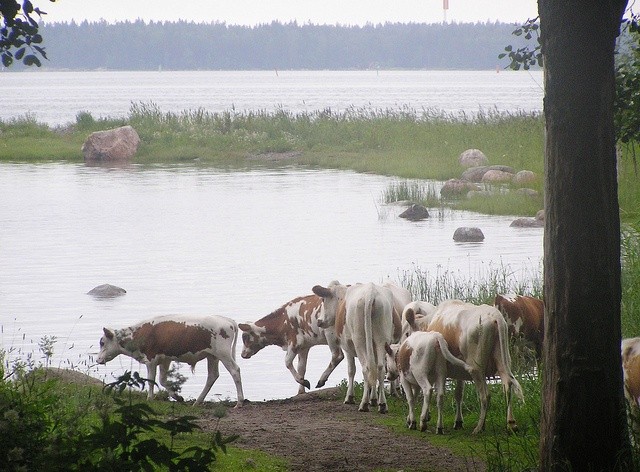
[383,330,483,436]
[398,299,527,435]
[237,292,345,396]
[363,282,411,397]
[621,335,639,412]
[495,293,544,373]
[311,279,403,415]
[95,310,246,411]
